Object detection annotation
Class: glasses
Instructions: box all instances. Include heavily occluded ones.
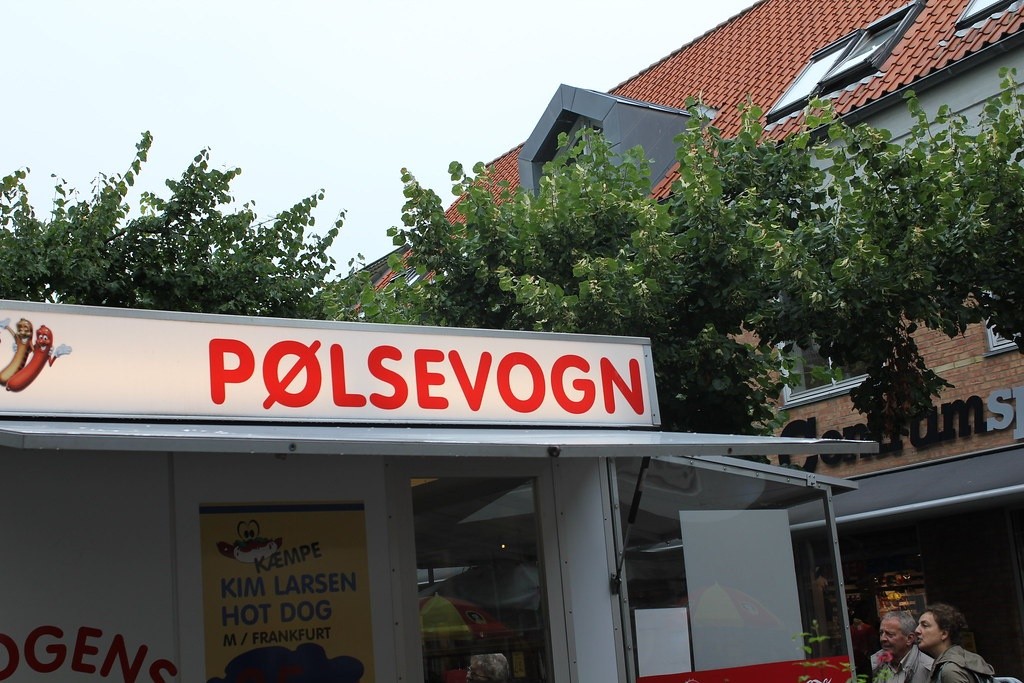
[467,666,488,680]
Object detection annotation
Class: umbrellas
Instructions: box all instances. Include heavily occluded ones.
[419,592,513,674]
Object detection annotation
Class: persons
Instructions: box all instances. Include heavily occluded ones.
[850,605,995,683]
[466,653,509,683]
